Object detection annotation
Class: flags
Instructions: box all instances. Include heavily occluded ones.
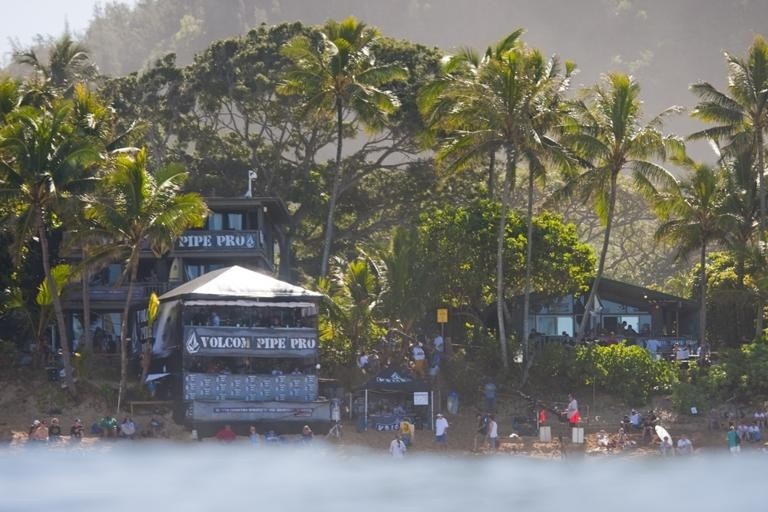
[248,167,259,180]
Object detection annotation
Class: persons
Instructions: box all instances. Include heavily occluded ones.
[388,432,406,458]
[398,417,412,446]
[29,414,164,446]
[726,407,768,453]
[595,409,694,456]
[565,391,579,428]
[434,413,449,455]
[528,321,702,359]
[355,331,444,377]
[189,306,315,445]
[468,413,489,453]
[479,415,498,455]
